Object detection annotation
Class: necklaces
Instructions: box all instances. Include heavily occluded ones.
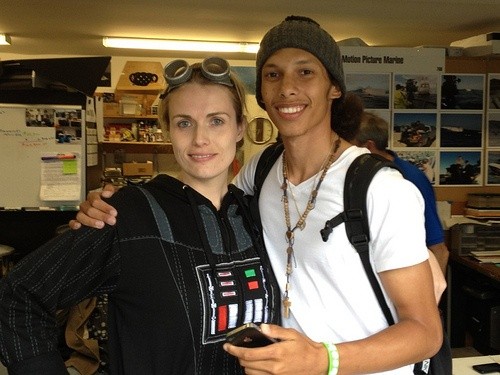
[281,134,341,318]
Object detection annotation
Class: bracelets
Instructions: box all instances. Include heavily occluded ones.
[320,341,339,375]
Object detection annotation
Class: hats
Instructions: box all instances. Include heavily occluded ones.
[256,15,345,113]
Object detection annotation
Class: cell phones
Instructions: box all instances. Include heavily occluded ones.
[225,322,279,349]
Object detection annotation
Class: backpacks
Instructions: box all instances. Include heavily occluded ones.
[254,142,452,375]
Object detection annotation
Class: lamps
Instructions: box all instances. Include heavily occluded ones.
[103,37,261,54]
[0,33,12,46]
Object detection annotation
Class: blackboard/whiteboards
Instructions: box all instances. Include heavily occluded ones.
[0,104,88,213]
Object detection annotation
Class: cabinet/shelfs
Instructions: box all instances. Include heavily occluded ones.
[87,92,180,200]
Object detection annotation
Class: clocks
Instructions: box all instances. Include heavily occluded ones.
[247,117,273,144]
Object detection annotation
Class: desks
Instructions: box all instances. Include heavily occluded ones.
[446,251,500,352]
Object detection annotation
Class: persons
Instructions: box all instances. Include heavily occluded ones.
[0,62,282,375]
[69,15,443,375]
[342,91,453,375]
